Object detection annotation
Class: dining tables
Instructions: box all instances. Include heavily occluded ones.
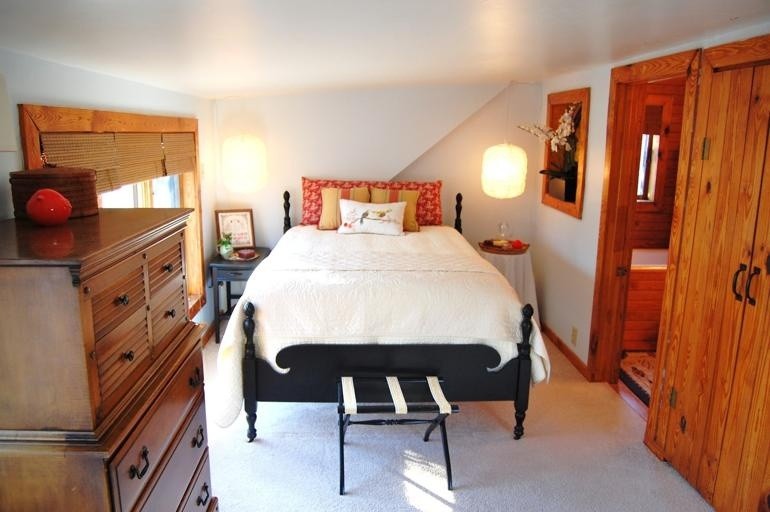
[215,231,234,260]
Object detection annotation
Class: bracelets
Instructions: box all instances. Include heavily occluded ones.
[237,249,256,259]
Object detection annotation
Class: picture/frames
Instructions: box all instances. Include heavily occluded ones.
[215,209,255,249]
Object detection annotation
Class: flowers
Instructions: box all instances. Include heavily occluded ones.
[517,102,580,178]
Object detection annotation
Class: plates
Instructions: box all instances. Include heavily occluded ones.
[650,34,769,511]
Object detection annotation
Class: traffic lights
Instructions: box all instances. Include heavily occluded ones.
[300,175,443,236]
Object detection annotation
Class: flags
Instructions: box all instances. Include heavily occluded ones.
[548,175,576,201]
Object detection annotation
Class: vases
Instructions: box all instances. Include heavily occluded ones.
[548,175,576,201]
[517,102,580,178]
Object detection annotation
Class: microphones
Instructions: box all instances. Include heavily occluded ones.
[337,376,459,495]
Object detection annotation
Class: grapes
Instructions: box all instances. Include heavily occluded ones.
[208,247,268,344]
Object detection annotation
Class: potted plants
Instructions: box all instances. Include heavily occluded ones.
[215,209,255,249]
[215,231,234,260]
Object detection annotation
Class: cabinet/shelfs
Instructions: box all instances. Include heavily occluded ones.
[650,34,769,511]
[1,208,196,445]
[4,326,218,512]
[497,222,514,247]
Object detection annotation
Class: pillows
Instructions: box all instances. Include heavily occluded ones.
[300,175,443,236]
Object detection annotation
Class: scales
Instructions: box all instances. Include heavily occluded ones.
[632,248,670,267]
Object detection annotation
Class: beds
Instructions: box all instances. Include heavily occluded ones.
[243,192,532,442]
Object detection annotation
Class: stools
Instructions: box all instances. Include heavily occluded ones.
[337,376,459,495]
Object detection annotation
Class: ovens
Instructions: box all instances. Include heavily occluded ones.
[243,192,532,442]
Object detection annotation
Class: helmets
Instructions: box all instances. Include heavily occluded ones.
[229,254,260,261]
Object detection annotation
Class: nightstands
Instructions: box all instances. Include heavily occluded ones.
[208,247,268,344]
